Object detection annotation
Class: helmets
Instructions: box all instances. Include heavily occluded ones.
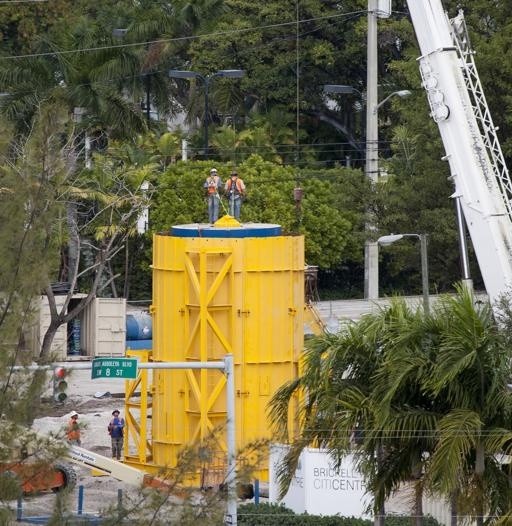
[230,171,239,176]
[69,410,78,417]
[111,410,120,415]
[210,168,217,173]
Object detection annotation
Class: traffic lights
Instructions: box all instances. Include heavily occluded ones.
[53,365,67,402]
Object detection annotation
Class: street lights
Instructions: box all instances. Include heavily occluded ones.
[377,232,430,319]
[168,68,247,157]
[322,83,412,298]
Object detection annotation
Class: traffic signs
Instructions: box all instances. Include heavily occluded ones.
[91,357,137,379]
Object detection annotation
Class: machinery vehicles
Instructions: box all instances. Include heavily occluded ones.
[0,421,212,508]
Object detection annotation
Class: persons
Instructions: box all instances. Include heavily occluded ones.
[67,410,81,447]
[225,170,247,221]
[202,168,222,225]
[107,408,126,460]
